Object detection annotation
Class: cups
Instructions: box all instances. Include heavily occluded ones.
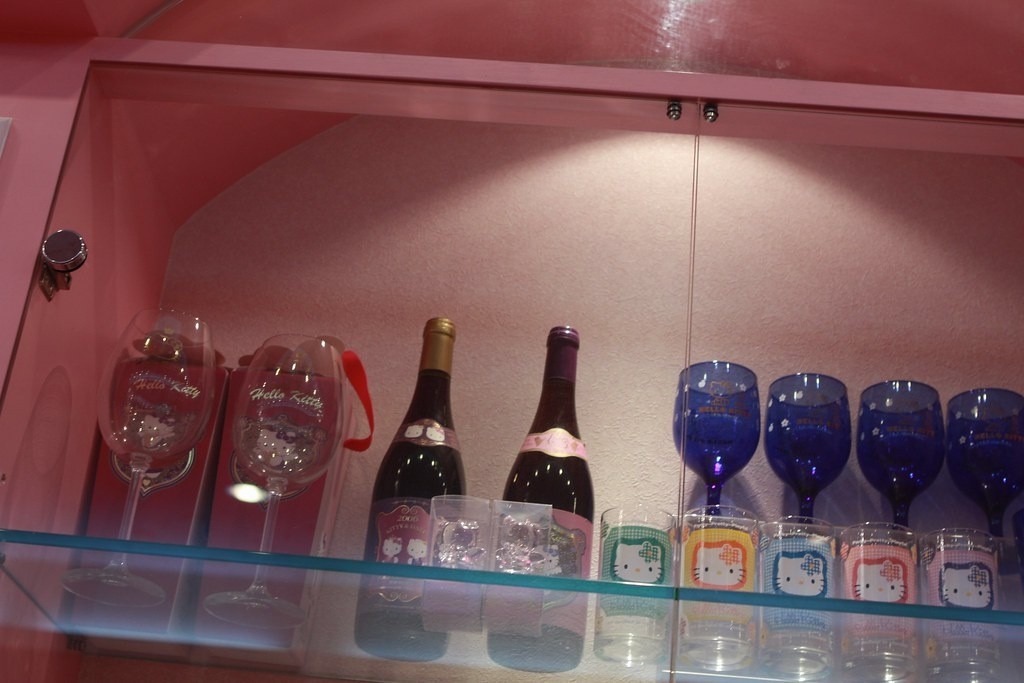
[674,505,756,672]
[600,506,674,665]
[758,516,836,681]
[922,529,1002,683]
[840,523,918,683]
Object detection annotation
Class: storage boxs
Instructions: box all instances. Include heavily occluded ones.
[74,356,234,656]
[484,500,554,637]
[420,495,493,635]
[189,368,357,670]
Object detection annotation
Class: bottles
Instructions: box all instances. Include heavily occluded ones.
[355,317,465,662]
[486,327,594,672]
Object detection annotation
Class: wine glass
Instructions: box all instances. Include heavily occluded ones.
[766,373,850,535]
[856,381,943,538]
[201,335,343,637]
[57,307,214,612]
[673,362,761,519]
[943,390,1023,573]
